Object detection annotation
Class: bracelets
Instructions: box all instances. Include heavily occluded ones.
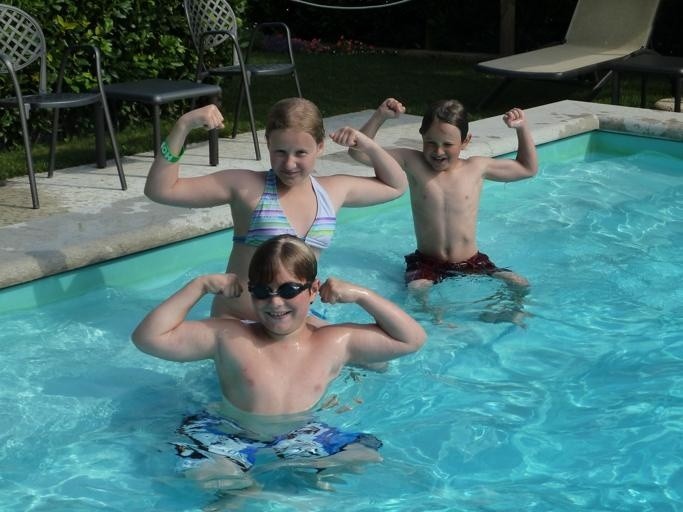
[159,137,185,163]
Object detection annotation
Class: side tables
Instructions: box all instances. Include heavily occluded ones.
[612,53,683,112]
[89,77,223,168]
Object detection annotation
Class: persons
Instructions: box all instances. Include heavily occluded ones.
[347,97,539,331]
[130,233,429,512]
[143,95,410,327]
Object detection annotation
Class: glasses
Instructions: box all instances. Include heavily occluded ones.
[248,282,311,299]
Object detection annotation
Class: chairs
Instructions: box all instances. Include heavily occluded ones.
[0,3,127,209]
[474,0,661,110]
[183,0,302,159]
